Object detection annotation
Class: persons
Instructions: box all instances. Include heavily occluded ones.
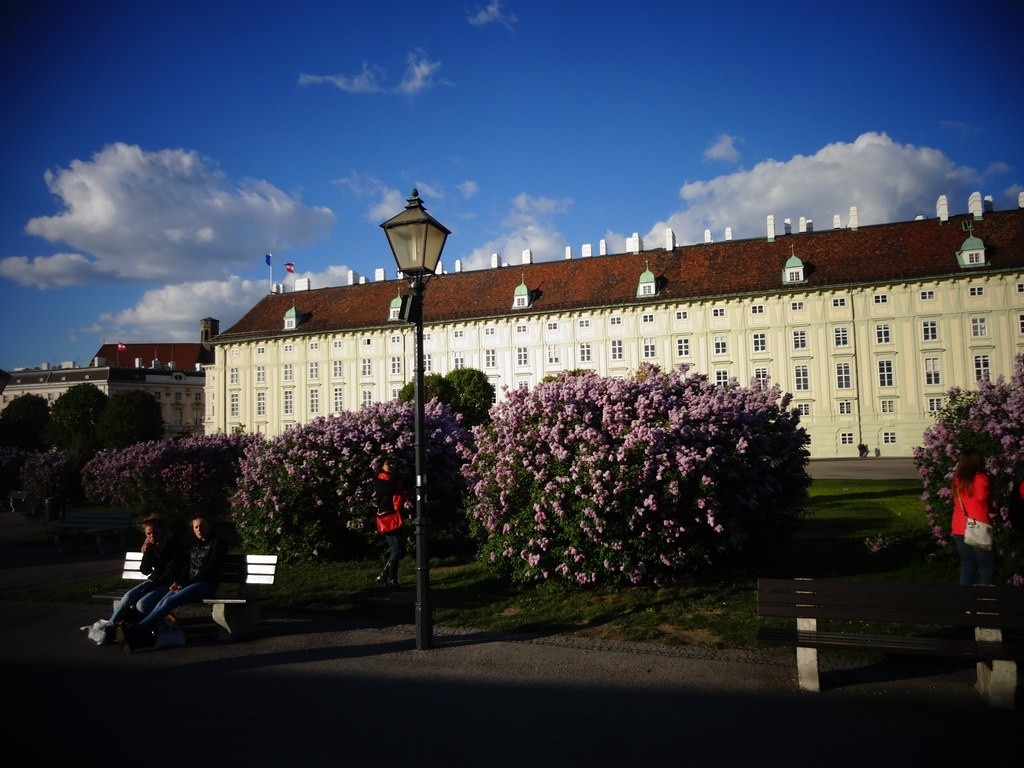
[79,514,189,646]
[116,514,222,656]
[372,457,413,593]
[950,448,999,614]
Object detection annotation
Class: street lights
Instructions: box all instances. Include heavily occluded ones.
[378,186,451,651]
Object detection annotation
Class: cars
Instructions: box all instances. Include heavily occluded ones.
[170,431,193,441]
[38,488,141,522]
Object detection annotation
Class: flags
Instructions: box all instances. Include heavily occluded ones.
[285,263,294,272]
[265,254,271,266]
[118,342,127,351]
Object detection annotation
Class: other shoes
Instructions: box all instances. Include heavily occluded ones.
[105,624,143,657]
[87,619,118,646]
[375,575,392,591]
[389,579,405,592]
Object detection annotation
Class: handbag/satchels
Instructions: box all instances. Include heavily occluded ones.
[376,509,403,536]
[963,518,994,552]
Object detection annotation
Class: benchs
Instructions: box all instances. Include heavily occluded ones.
[90,552,279,642]
[756,577,1023,708]
